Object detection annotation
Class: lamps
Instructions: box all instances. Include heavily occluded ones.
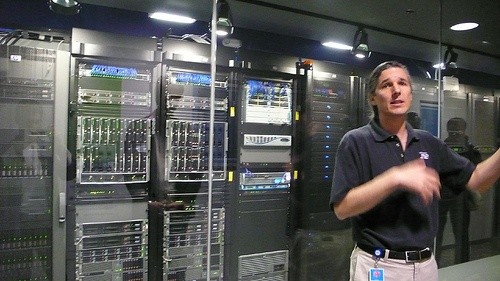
[208,0,234,36]
[352,26,372,60]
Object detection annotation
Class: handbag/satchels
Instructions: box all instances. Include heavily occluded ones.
[465,190,482,211]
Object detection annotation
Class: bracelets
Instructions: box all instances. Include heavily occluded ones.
[147,200,152,205]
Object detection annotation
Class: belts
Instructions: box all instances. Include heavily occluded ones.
[357,242,433,263]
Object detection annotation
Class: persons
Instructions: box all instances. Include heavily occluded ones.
[436,118,485,268]
[329,61,500,281]
[122,72,207,281]
[0,97,76,281]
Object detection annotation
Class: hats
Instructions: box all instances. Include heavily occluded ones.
[447,118,466,131]
